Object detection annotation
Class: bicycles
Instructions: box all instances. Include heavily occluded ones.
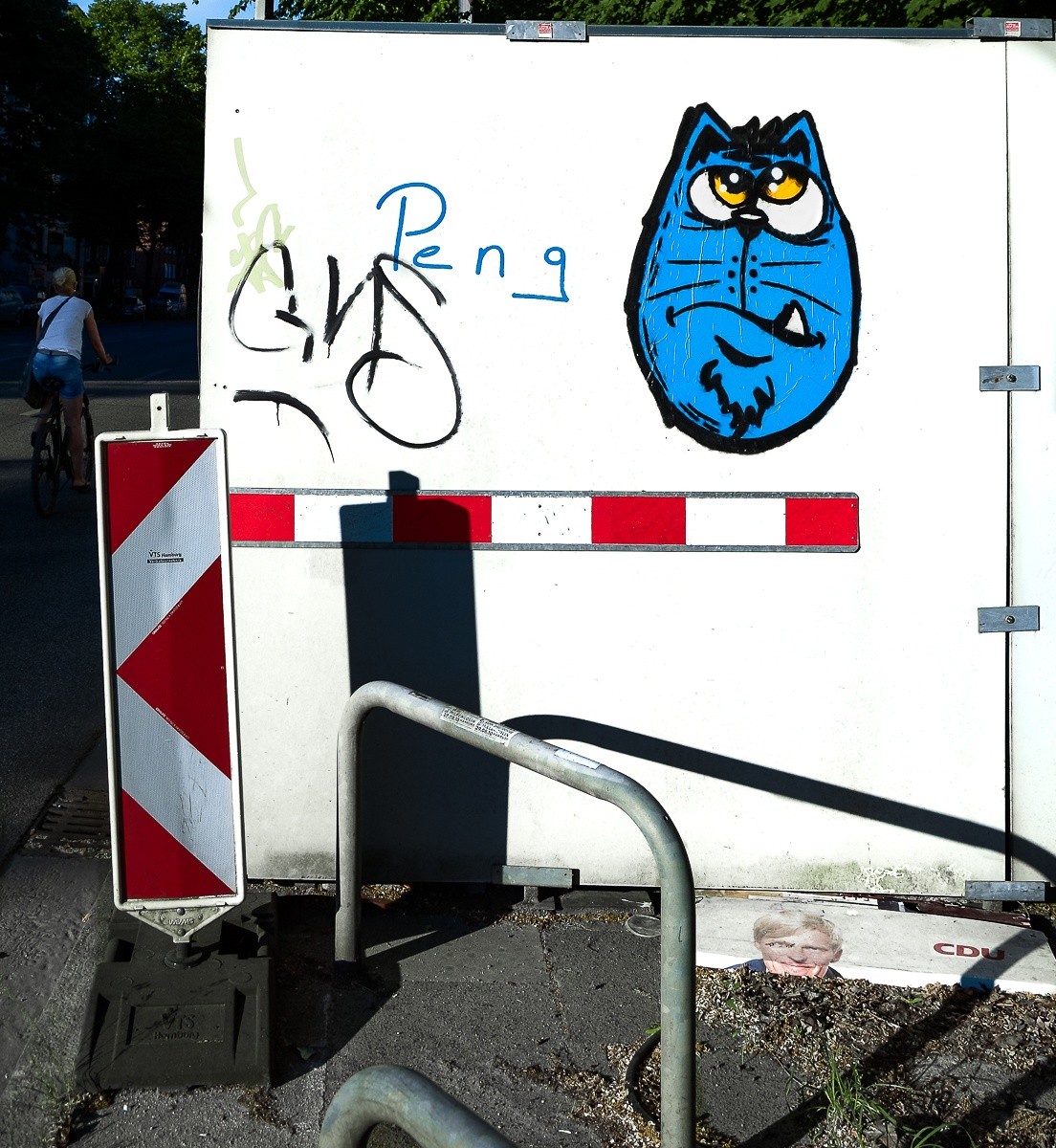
[28,354,119,521]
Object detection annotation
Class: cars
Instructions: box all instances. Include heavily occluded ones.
[115,295,147,318]
[0,283,36,329]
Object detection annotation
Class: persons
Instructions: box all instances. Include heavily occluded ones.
[722,905,844,978]
[30,265,114,489]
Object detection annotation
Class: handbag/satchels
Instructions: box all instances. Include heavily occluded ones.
[21,348,42,410]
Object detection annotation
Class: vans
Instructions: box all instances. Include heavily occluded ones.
[154,281,188,319]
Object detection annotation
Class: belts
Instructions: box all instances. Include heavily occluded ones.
[38,349,69,355]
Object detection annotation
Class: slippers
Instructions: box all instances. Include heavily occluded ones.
[71,481,90,488]
[30,431,38,446]
